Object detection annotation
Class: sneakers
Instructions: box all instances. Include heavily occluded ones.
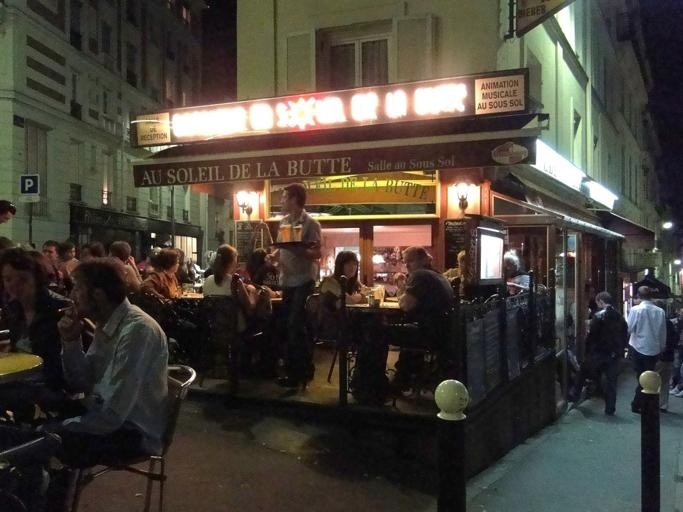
[669,384,683,397]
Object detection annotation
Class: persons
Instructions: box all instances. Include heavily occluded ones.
[668,308,683,398]
[652,299,680,410]
[0,183,533,510]
[626,284,667,413]
[568,291,629,415]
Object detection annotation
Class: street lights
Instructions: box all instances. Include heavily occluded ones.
[653,222,682,295]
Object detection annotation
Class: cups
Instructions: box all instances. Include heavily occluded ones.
[279,223,292,244]
[368,285,386,309]
[292,223,303,242]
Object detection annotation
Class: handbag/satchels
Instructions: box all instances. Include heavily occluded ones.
[306,290,340,340]
